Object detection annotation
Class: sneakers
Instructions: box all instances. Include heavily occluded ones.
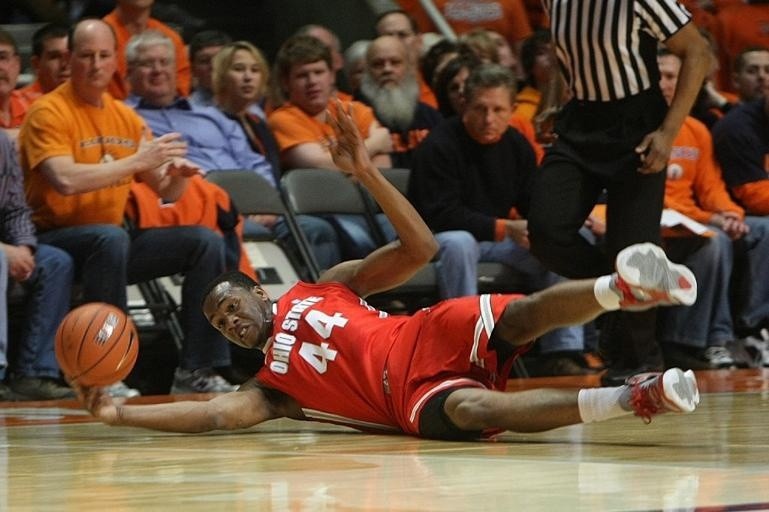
[1,372,76,400]
[544,350,613,377]
[729,328,769,368]
[600,361,665,389]
[698,344,742,370]
[619,369,701,425]
[608,242,699,311]
[170,366,240,396]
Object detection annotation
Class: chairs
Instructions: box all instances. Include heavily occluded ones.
[1,18,528,378]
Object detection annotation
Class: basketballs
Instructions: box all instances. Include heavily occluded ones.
[55,303,139,387]
